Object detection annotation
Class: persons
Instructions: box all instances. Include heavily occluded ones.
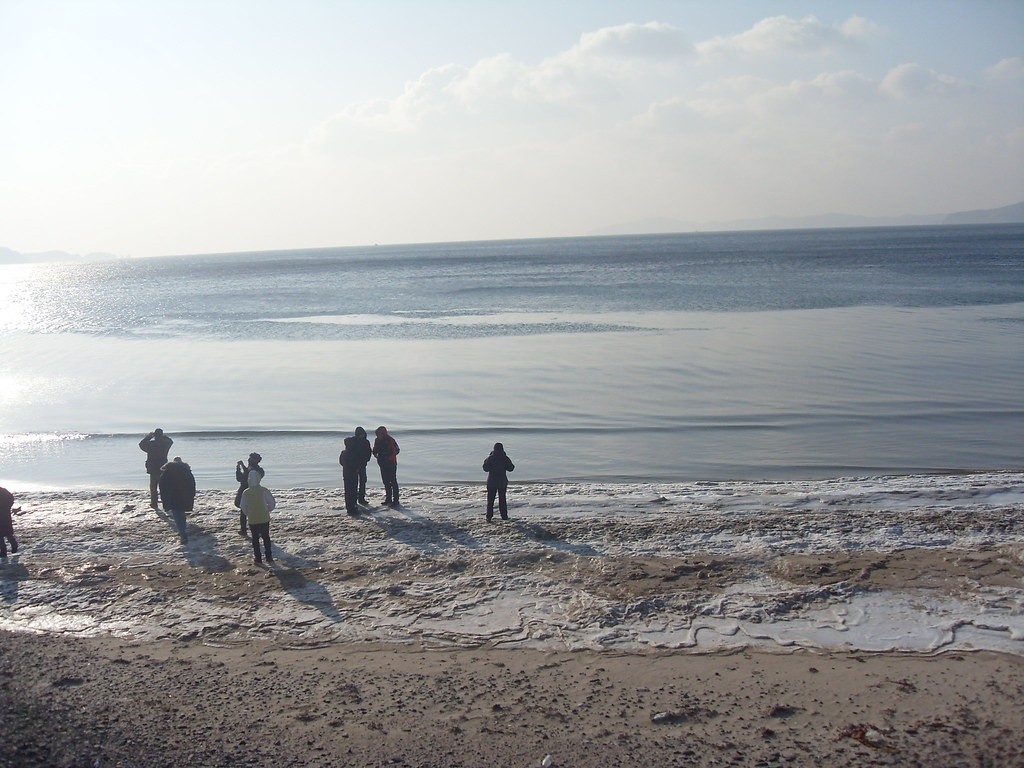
[373,426,400,507]
[0,487,18,558]
[241,471,276,563]
[158,457,196,544]
[234,452,265,535]
[483,442,515,522]
[139,428,173,508]
[339,428,373,513]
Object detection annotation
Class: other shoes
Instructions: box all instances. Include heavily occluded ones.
[0,552,7,557]
[381,501,392,504]
[347,508,363,514]
[12,545,18,553]
[238,529,247,535]
[359,500,369,504]
[393,500,399,504]
